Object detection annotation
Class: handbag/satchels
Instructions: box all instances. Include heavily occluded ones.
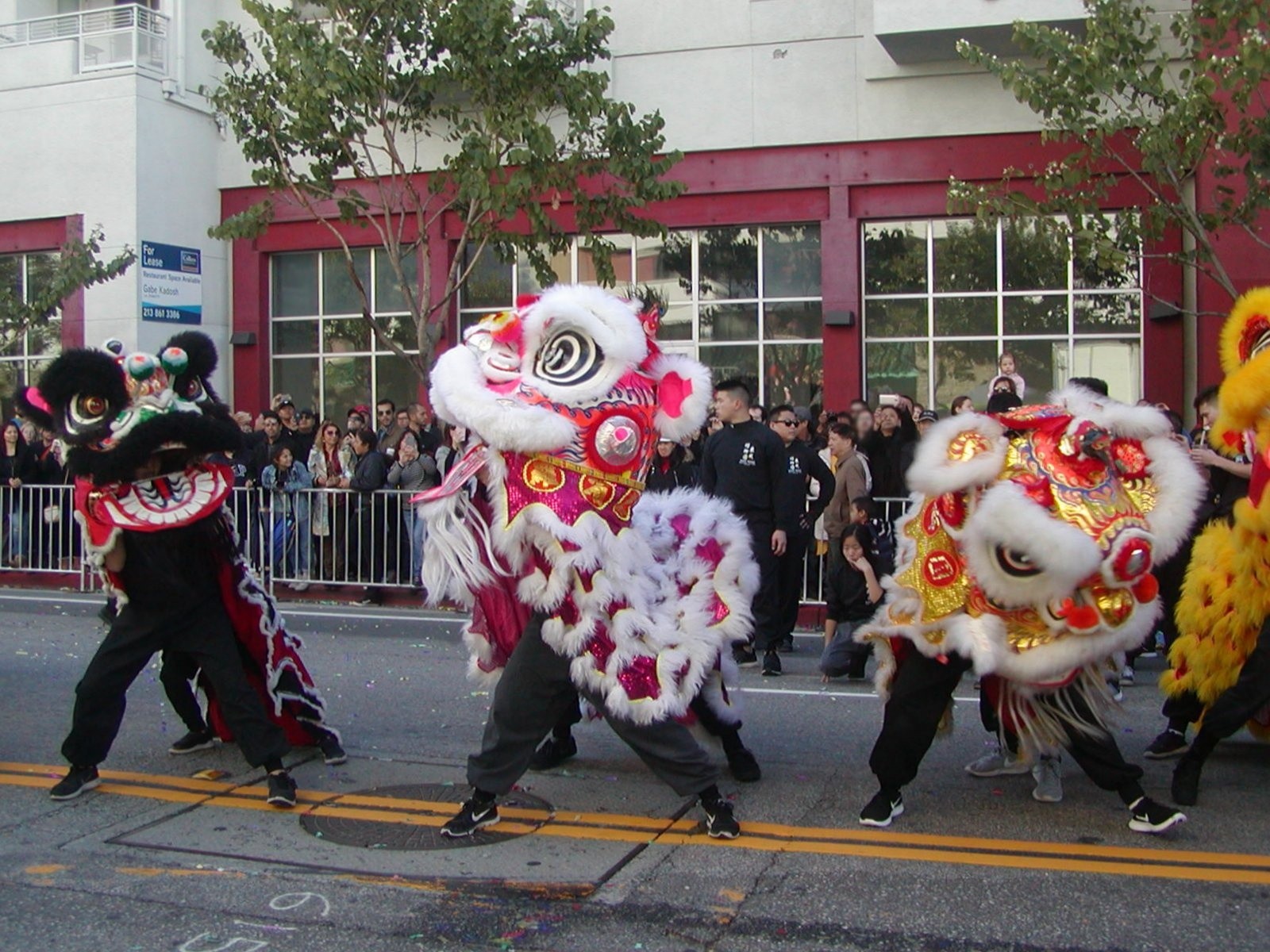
[44,505,60,524]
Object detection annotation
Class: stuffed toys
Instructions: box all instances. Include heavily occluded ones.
[16,330,343,750]
[412,282,759,757]
[1150,286,1270,743]
[848,380,1210,757]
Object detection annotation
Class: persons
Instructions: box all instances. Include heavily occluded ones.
[1144,610,1270,806]
[48,500,299,811]
[158,646,347,766]
[205,394,478,614]
[641,352,1025,686]
[0,401,94,576]
[857,638,1188,834]
[1068,374,1255,699]
[526,675,763,781]
[436,604,742,837]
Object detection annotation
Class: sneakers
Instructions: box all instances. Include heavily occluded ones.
[860,788,904,826]
[1120,644,1158,685]
[49,765,102,799]
[319,739,347,764]
[969,743,1064,803]
[266,772,297,808]
[706,799,741,838]
[440,797,499,838]
[531,736,577,773]
[172,729,214,754]
[728,750,762,783]
[731,636,795,675]
[1096,728,1202,834]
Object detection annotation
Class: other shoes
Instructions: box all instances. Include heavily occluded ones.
[848,650,869,679]
[0,545,90,576]
[97,607,116,625]
[261,559,424,607]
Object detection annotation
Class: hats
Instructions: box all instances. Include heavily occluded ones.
[348,405,371,421]
[792,405,811,423]
[278,400,295,412]
[917,410,938,424]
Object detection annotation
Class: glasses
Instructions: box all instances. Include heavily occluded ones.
[297,415,313,419]
[348,416,364,424]
[994,387,1010,393]
[325,431,339,436]
[774,418,801,428]
[378,410,392,417]
